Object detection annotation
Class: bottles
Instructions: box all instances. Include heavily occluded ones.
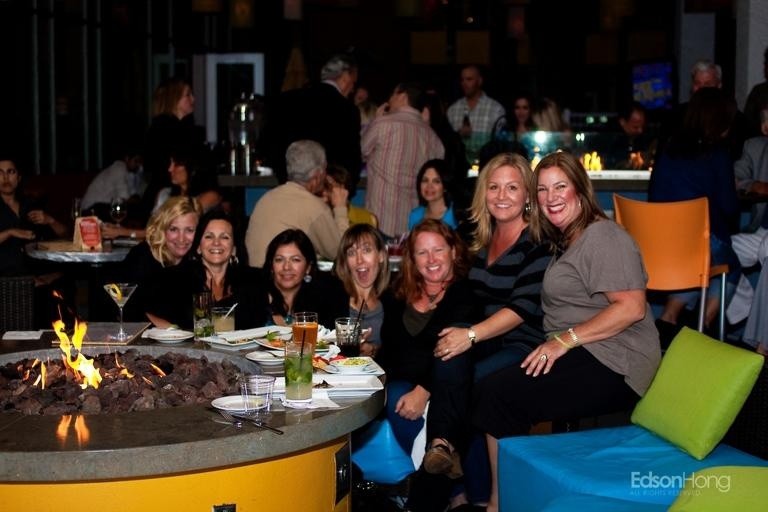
[460,106,472,137]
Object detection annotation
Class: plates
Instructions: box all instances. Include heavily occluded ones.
[148,330,194,345]
[317,260,334,271]
[198,324,386,417]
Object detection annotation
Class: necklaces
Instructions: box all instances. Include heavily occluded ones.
[360,293,374,313]
[420,281,445,311]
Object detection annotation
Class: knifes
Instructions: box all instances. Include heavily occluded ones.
[230,414,283,436]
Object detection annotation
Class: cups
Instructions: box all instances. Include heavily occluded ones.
[290,310,319,356]
[211,308,235,332]
[71,198,95,219]
[387,240,404,271]
[192,292,215,349]
[334,316,363,357]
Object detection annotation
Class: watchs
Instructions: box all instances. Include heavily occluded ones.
[467,325,475,345]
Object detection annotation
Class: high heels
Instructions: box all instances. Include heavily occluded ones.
[420,438,462,480]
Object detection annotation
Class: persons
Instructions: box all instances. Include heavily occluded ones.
[193,210,267,330]
[141,57,582,277]
[328,224,399,350]
[423,152,559,512]
[483,153,658,511]
[257,228,345,329]
[52,90,71,167]
[0,160,73,310]
[603,39,767,354]
[383,222,478,438]
[82,147,140,225]
[106,195,200,326]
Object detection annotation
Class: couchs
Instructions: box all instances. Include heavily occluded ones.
[494,423,766,512]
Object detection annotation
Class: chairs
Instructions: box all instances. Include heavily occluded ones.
[0,275,37,331]
[609,189,733,344]
[345,205,378,231]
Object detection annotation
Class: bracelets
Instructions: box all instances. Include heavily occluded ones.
[567,322,580,346]
[554,333,572,349]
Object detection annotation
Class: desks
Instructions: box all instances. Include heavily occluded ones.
[215,167,652,218]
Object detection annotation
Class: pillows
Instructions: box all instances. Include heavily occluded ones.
[665,464,766,512]
[626,322,766,462]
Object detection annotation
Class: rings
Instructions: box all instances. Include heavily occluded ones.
[445,348,449,354]
[541,354,548,361]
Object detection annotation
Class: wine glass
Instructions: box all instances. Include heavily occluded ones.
[109,196,129,226]
[104,283,138,339]
